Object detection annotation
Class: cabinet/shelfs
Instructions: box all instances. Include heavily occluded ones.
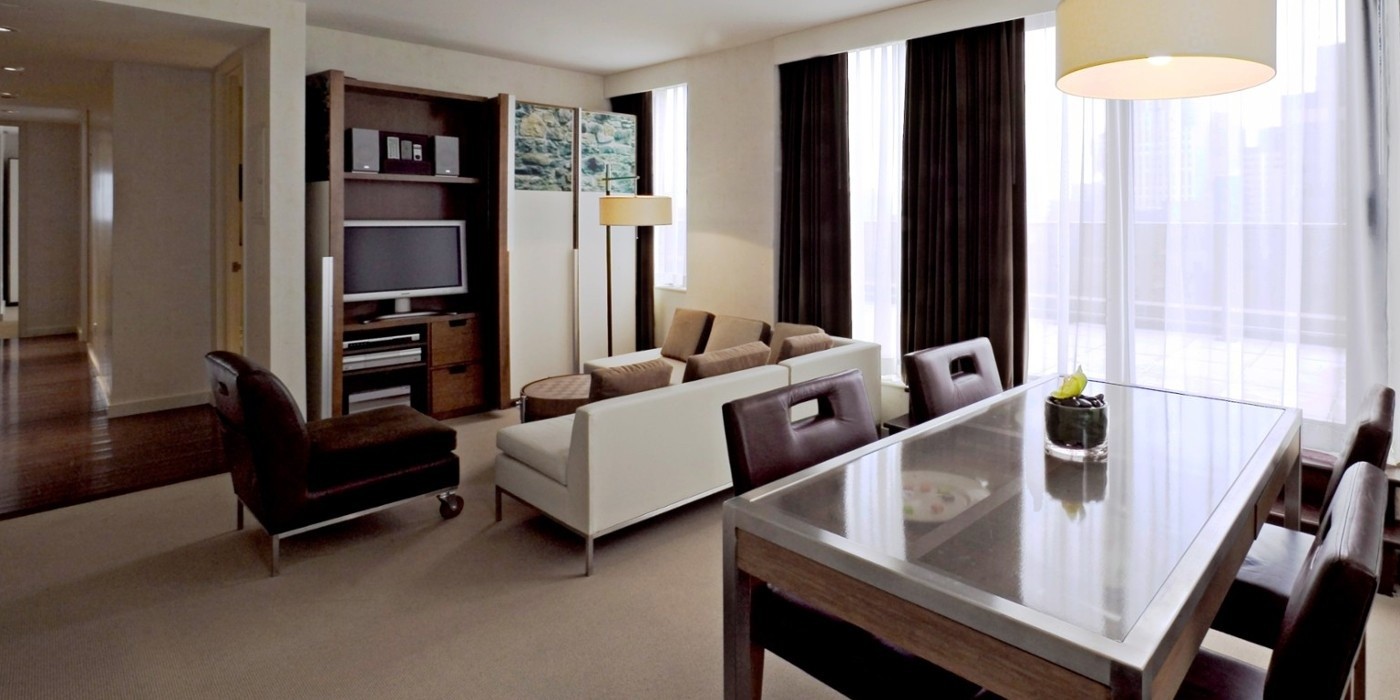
[306,70,510,424]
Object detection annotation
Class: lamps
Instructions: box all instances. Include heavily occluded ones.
[1052,0,1280,103]
[596,166,671,359]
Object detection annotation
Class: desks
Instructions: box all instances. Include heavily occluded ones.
[720,371,1306,700]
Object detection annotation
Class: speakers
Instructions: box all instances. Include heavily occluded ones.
[344,127,379,174]
[429,136,459,177]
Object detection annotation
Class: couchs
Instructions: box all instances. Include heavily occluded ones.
[206,350,463,579]
[493,313,883,575]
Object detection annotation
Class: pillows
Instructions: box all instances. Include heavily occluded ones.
[776,332,835,363]
[589,358,674,407]
[660,309,716,362]
[683,341,772,385]
[768,323,825,366]
[704,316,772,354]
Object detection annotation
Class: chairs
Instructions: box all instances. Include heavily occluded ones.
[1172,461,1390,700]
[1206,382,1397,698]
[719,368,914,700]
[902,335,1007,436]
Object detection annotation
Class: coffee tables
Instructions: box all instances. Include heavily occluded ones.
[516,373,594,426]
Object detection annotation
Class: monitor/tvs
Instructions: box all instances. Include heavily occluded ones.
[343,219,468,302]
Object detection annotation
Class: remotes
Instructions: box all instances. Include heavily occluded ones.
[443,311,457,315]
[386,134,423,161]
[355,316,371,325]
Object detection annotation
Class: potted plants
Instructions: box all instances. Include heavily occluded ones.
[1044,367,1110,465]
